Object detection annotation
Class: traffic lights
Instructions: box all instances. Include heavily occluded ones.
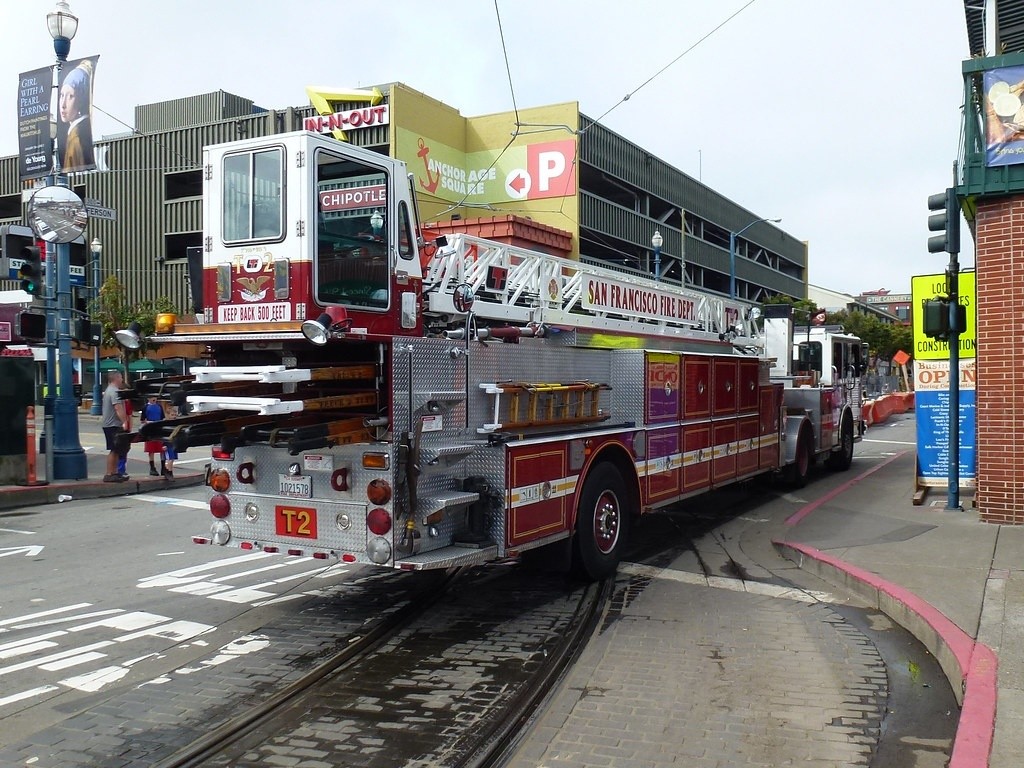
[927,187,962,253]
[19,245,43,296]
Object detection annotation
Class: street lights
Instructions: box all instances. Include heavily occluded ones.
[90,236,104,416]
[652,228,664,283]
[731,218,783,311]
[40,0,81,419]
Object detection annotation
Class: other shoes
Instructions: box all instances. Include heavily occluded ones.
[118,473,129,481]
[150,469,158,476]
[104,473,123,483]
[161,469,166,475]
[165,471,176,483]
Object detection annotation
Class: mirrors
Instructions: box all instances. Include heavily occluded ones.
[28,184,89,244]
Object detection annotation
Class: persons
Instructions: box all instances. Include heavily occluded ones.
[140,397,178,478]
[101,370,132,483]
[59,60,95,169]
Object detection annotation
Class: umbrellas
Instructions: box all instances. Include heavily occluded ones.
[128,358,171,375]
[169,360,204,375]
[85,357,128,375]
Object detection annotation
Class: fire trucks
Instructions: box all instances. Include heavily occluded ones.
[103,129,874,586]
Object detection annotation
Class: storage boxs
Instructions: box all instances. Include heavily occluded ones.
[80,398,94,408]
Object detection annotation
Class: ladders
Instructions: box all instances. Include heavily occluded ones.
[102,364,375,457]
[476,382,612,433]
